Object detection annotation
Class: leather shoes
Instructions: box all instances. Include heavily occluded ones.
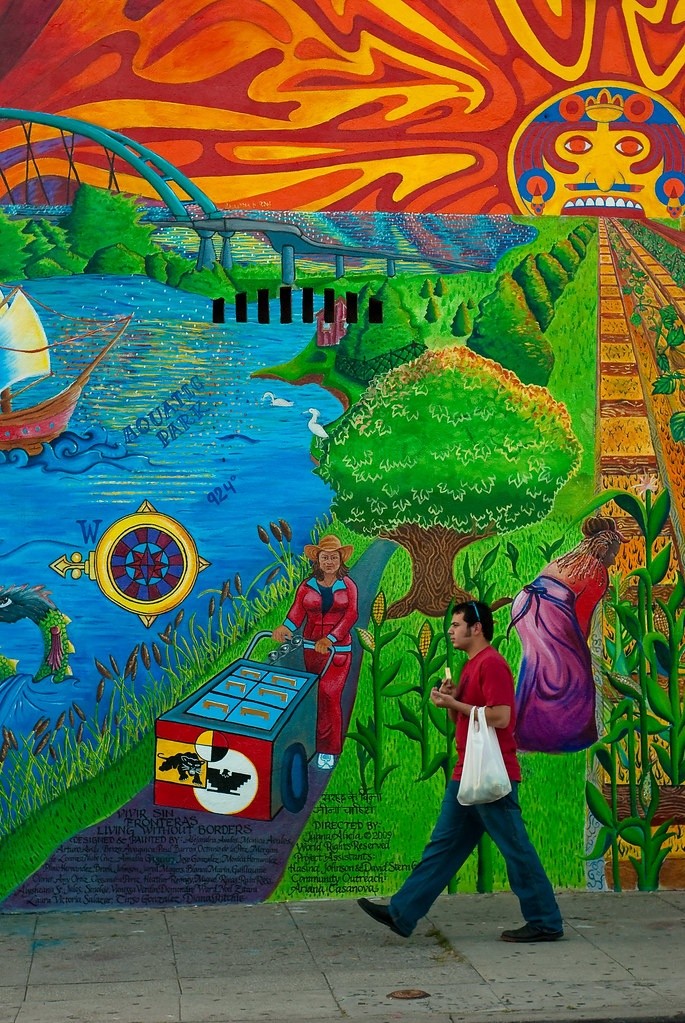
[357,898,413,938]
[501,923,564,942]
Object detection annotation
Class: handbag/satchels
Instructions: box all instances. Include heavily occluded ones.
[456,706,512,807]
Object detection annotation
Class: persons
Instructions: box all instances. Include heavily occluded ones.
[356,600,564,944]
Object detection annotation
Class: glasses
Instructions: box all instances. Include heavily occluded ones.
[468,600,481,622]
